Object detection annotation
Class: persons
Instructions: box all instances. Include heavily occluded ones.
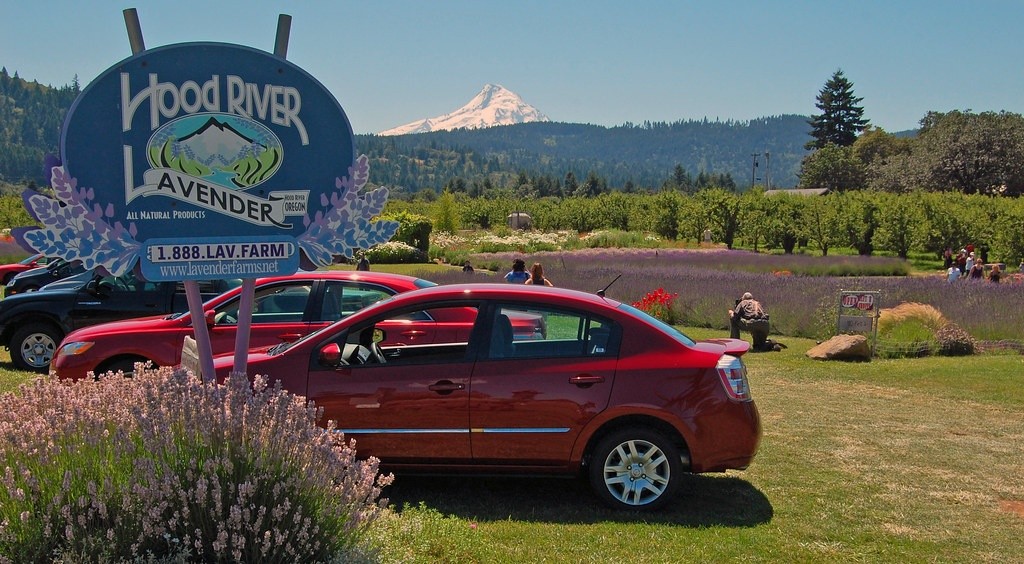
[504,258,531,312]
[944,241,975,279]
[728,292,781,352]
[966,258,984,281]
[356,253,370,292]
[462,260,475,274]
[987,264,1005,285]
[524,262,554,340]
[944,261,961,286]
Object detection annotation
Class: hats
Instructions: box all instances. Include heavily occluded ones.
[952,262,957,266]
[742,292,753,300]
[970,252,975,255]
[961,249,966,252]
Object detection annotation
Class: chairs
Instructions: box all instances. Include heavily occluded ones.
[491,314,515,358]
[322,292,340,321]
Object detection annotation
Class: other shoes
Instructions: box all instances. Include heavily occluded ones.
[771,339,781,352]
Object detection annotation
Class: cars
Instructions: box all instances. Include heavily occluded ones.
[4,257,87,299]
[0,267,242,375]
[48,270,546,396]
[0,253,48,285]
[195,282,766,512]
